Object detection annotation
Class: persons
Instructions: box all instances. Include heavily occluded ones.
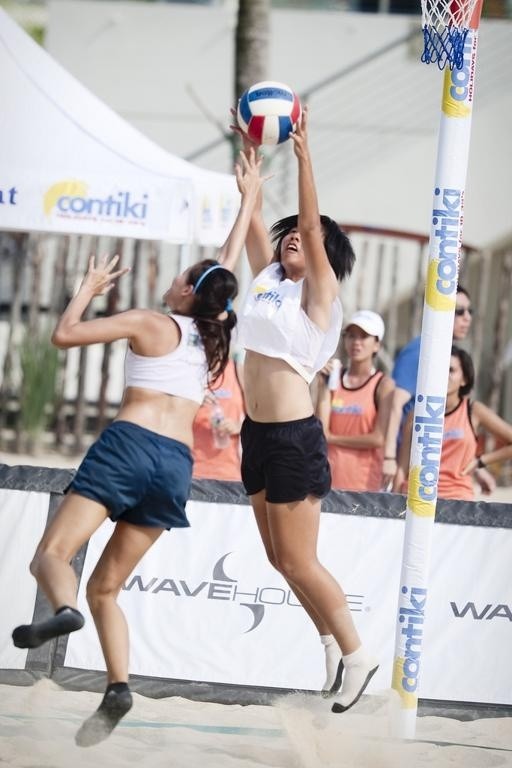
[11,146,274,747]
[315,310,394,493]
[390,345,512,502]
[231,105,380,713]
[191,311,244,481]
[382,285,471,488]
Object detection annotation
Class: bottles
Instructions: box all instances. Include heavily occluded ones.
[210,407,228,451]
[325,357,345,392]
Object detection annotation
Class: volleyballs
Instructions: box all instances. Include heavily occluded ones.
[236,81,301,149]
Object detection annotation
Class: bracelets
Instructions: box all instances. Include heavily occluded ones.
[384,457,396,460]
[477,456,485,468]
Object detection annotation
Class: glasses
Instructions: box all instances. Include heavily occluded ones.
[454,307,473,317]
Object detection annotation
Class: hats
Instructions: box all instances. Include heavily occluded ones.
[341,307,386,340]
